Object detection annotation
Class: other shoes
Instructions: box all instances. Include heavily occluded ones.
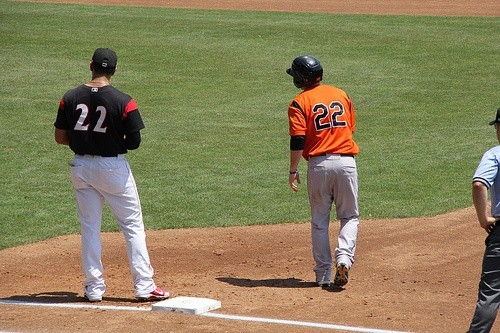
[135,286,169,301]
[334,263,348,287]
[84,292,102,302]
[318,280,331,286]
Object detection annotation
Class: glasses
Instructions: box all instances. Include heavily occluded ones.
[494,123,500,128]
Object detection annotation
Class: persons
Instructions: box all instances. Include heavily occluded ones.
[464,108,500,332]
[54,48,169,300]
[285,55,360,287]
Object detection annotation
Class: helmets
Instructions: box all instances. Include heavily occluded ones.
[286,55,322,87]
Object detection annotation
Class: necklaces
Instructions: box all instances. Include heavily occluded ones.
[89,80,109,83]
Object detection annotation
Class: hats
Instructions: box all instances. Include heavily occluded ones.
[92,47,118,68]
[488,107,500,125]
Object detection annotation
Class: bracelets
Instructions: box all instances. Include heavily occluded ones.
[289,168,298,174]
